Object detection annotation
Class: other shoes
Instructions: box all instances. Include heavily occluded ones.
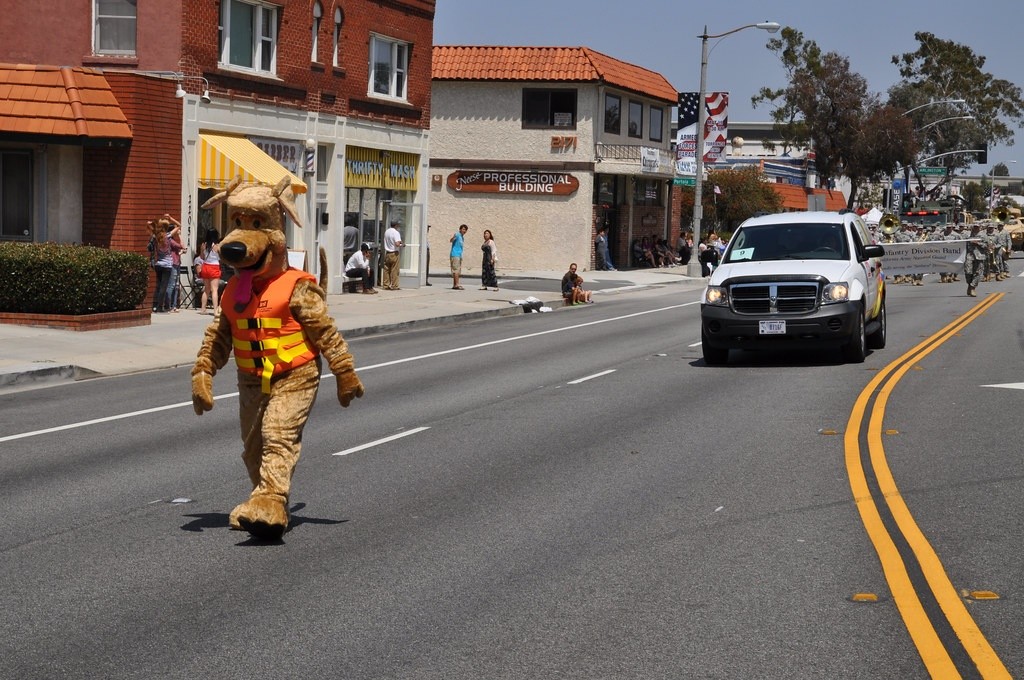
[453,285,464,290]
[596,268,617,271]
[363,288,378,294]
[152,306,180,313]
[384,286,400,290]
[426,283,432,286]
[675,257,682,261]
[479,287,487,290]
[648,263,680,268]
[201,310,219,315]
[493,287,499,291]
[572,299,594,306]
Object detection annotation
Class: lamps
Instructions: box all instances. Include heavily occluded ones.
[379,151,391,158]
[141,71,186,99]
[304,139,316,176]
[162,74,211,104]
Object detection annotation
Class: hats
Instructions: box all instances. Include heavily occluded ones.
[867,220,1004,231]
[361,243,374,252]
[428,225,431,227]
[915,186,919,189]
[391,217,401,225]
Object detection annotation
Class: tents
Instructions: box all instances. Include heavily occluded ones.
[861,208,884,227]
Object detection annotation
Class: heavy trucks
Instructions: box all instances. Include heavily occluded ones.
[901,193,975,224]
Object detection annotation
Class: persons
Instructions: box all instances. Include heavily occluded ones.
[562,263,583,304]
[595,228,606,270]
[870,220,1012,297]
[346,242,379,294]
[343,216,360,265]
[479,230,499,291]
[426,225,432,286]
[633,230,729,276]
[450,224,468,290]
[147,214,222,317]
[575,277,594,303]
[604,228,617,272]
[383,218,405,290]
[822,233,838,252]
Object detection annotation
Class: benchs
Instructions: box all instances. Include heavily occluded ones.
[343,275,363,293]
[631,250,660,268]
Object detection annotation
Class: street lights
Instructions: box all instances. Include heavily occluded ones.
[989,160,1017,217]
[886,99,976,211]
[686,19,782,278]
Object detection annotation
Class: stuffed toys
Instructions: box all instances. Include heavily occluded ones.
[191,176,364,544]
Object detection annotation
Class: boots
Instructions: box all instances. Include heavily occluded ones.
[984,271,1010,281]
[967,284,976,297]
[938,276,961,283]
[886,275,923,286]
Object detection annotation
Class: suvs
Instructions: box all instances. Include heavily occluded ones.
[697,209,889,368]
[990,206,1024,253]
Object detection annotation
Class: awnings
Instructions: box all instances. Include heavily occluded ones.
[197,132,307,194]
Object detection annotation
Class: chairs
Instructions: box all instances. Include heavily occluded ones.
[179,267,212,308]
